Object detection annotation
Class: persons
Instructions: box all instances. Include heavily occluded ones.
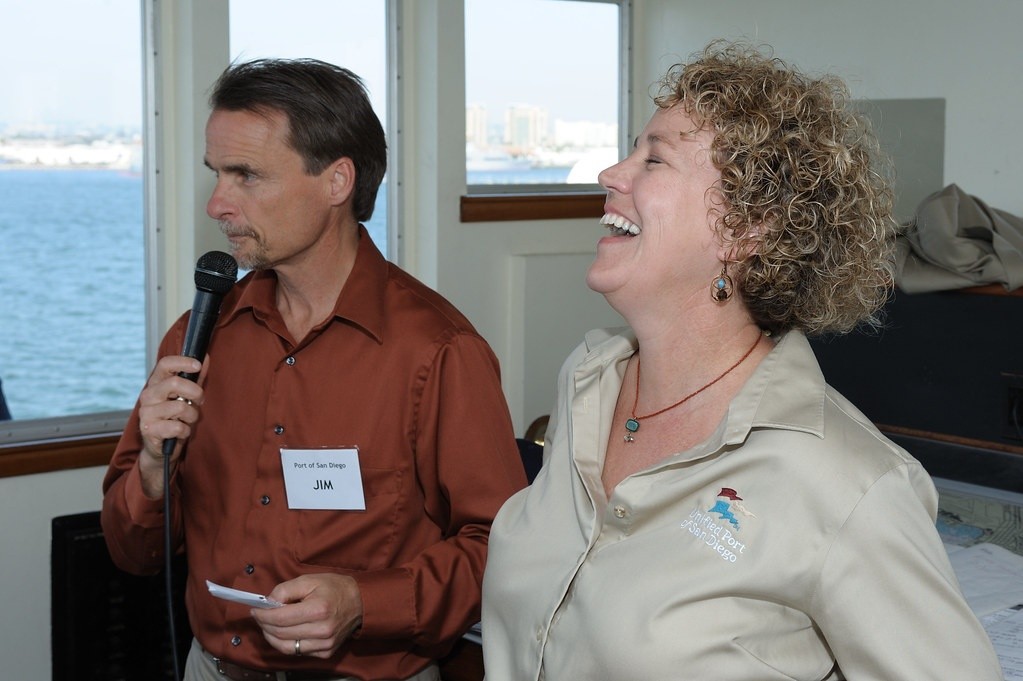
[102,55,529,681]
[481,36,1004,681]
[624,328,763,442]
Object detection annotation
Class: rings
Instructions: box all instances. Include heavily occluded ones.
[295,640,302,656]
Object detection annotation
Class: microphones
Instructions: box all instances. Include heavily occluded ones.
[162,251,239,456]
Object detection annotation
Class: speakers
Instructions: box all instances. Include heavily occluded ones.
[51,510,195,681]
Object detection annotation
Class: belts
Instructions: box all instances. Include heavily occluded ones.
[202,649,349,681]
